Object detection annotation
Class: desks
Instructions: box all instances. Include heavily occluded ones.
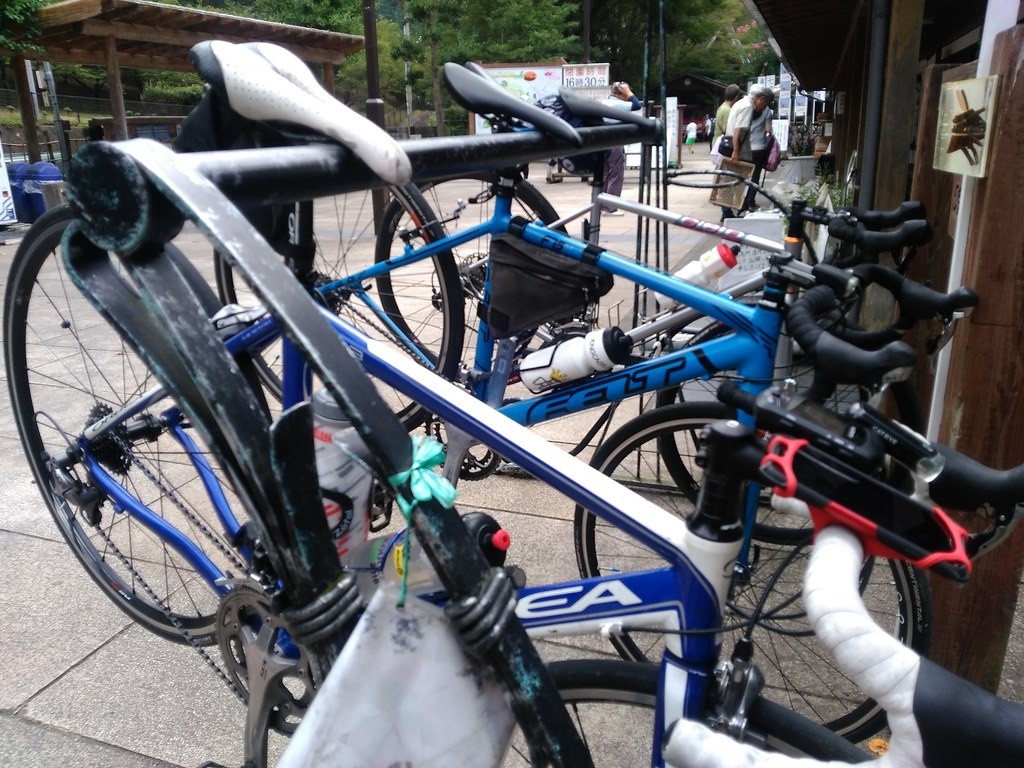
[723,210,787,247]
[778,156,816,198]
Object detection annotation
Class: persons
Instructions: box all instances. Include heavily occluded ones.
[600,82,642,218]
[744,83,770,211]
[705,114,714,151]
[711,84,741,158]
[719,88,773,219]
[684,118,696,155]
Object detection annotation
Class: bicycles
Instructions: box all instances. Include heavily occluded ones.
[0,23,1024,767]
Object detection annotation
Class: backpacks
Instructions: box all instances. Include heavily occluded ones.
[765,134,781,172]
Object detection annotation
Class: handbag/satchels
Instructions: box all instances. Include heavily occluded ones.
[718,135,742,158]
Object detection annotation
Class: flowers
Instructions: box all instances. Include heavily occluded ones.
[732,20,770,76]
[785,180,823,202]
[789,124,817,149]
